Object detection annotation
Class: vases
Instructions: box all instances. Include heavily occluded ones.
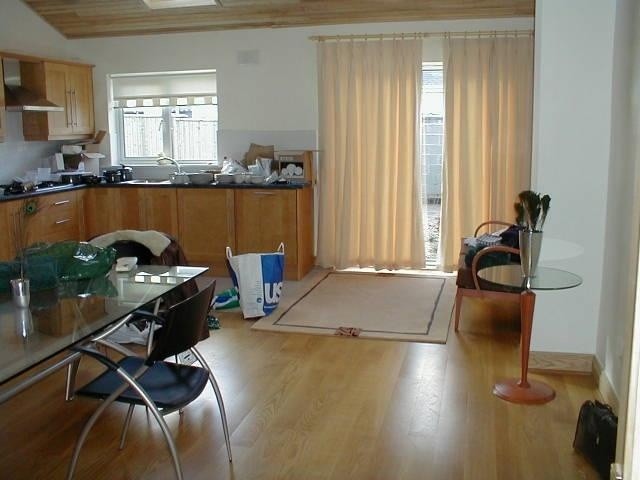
[519,230,543,278]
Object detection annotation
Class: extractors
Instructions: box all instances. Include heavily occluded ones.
[2,58,63,112]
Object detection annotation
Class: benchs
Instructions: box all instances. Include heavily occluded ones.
[455,221,521,332]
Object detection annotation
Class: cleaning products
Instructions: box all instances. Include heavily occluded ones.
[221,156,229,168]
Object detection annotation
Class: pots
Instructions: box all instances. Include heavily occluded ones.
[116,163,134,180]
[81,177,102,184]
[103,170,122,183]
[62,174,94,185]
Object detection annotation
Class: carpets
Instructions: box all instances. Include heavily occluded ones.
[250,267,459,344]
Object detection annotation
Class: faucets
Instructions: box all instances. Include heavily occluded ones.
[156,156,180,173]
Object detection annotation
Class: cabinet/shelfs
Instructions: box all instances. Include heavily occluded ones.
[87,188,120,241]
[120,188,178,245]
[0,197,38,261]
[235,188,314,281]
[21,58,96,141]
[176,188,236,278]
[38,191,78,248]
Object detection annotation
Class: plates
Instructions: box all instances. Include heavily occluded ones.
[222,156,229,168]
[214,174,234,184]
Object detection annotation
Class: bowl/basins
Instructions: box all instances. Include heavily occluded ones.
[189,173,213,185]
[200,170,221,183]
[168,173,190,184]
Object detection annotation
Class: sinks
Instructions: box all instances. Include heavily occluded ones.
[133,180,164,183]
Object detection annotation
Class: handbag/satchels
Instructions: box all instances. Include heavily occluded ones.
[225,242,285,318]
[573,400,618,480]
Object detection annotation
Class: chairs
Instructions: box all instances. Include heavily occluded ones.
[67,280,233,480]
[65,239,162,411]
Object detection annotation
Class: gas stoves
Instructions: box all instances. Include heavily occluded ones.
[37,181,62,189]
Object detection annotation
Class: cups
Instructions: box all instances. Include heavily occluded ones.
[256,157,273,177]
[9,279,30,306]
[244,171,253,183]
[251,176,266,185]
[235,173,244,184]
[248,165,257,176]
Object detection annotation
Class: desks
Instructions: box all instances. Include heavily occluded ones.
[477,266,583,404]
[0,264,209,415]
